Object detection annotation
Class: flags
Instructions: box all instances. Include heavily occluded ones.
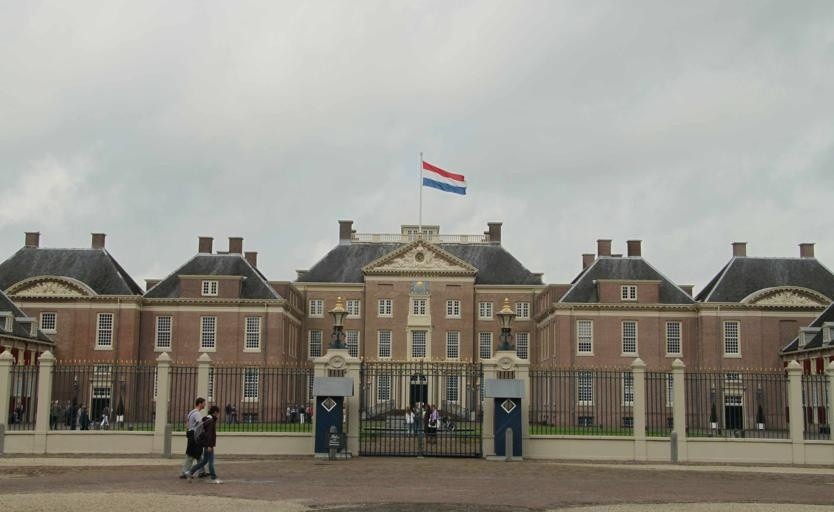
[423,161,465,195]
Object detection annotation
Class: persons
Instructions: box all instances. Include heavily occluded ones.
[179,398,210,478]
[50,399,109,430]
[286,404,313,424]
[230,406,238,424]
[225,402,232,425]
[405,403,439,443]
[185,406,222,485]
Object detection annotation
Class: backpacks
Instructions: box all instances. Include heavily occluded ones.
[194,423,205,446]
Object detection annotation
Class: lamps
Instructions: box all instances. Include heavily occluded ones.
[327,296,351,342]
[496,296,517,349]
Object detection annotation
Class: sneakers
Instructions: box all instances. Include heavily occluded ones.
[179,471,224,484]
